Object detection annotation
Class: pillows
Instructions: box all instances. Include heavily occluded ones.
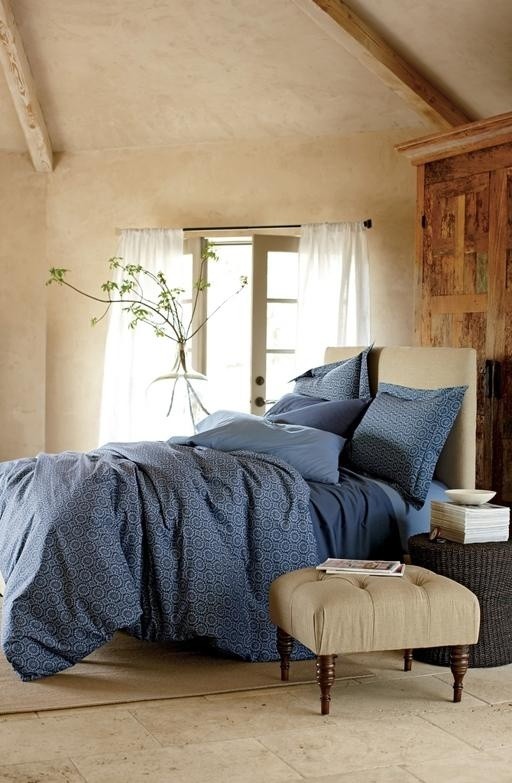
[341,381,469,510]
[288,341,375,402]
[264,393,371,434]
[167,411,348,489]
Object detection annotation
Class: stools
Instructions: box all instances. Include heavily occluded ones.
[408,532,512,668]
[270,562,481,714]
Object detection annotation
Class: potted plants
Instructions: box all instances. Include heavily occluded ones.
[44,240,249,439]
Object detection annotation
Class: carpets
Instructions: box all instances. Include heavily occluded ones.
[0,633,373,715]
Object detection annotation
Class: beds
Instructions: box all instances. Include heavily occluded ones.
[0,345,479,680]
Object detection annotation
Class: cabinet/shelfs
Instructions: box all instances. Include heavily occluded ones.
[394,110,512,507]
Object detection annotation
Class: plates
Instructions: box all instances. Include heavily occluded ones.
[445,489,497,506]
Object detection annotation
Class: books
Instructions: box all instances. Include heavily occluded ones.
[316,557,404,576]
[430,499,510,546]
